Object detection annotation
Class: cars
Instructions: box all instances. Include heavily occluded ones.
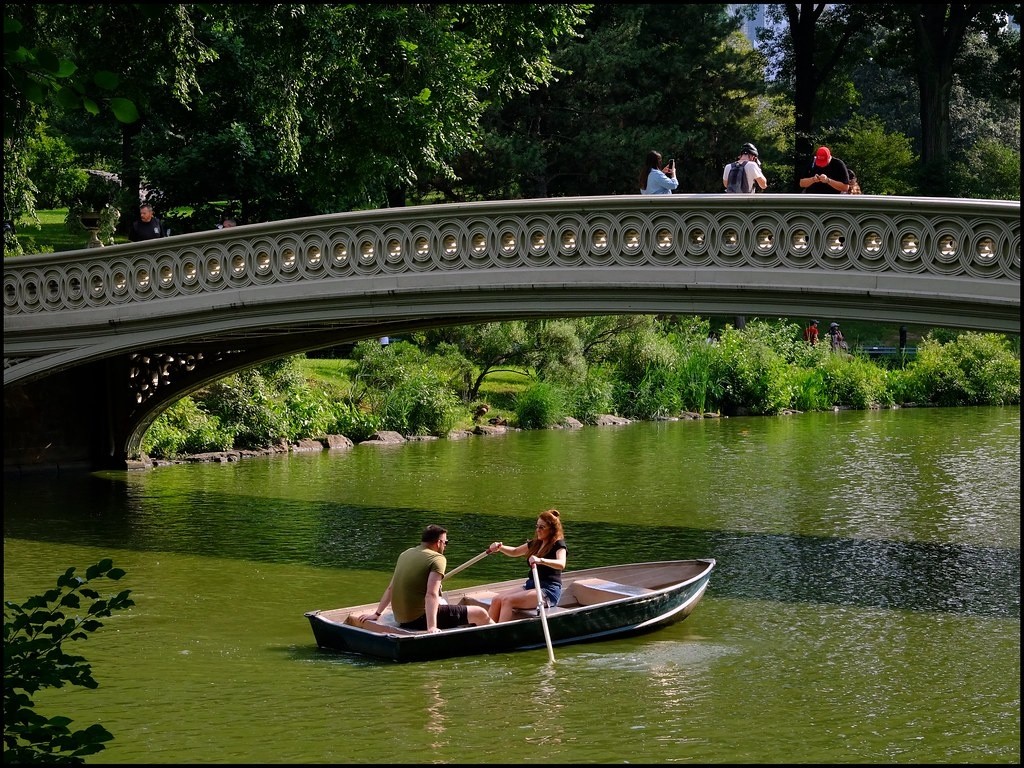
[65,168,172,233]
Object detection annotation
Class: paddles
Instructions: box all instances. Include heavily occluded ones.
[531,562,555,662]
[440,542,502,580]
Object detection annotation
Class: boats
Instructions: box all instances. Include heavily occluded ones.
[303,555,718,661]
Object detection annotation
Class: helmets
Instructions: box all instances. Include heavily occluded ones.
[740,143,759,156]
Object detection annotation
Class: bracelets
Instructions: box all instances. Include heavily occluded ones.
[375,611,381,616]
[828,179,831,184]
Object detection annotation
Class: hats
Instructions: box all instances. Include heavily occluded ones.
[830,322,840,327]
[815,147,830,166]
[848,169,855,180]
[810,319,820,324]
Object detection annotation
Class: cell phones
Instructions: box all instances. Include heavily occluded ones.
[668,159,675,171]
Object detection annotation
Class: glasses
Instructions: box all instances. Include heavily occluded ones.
[223,226,226,228]
[535,525,551,530]
[441,540,448,545]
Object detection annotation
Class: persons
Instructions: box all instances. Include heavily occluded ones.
[842,170,861,194]
[487,509,567,624]
[640,150,680,195]
[799,146,849,194]
[379,336,389,348]
[802,319,820,343]
[824,323,847,344]
[223,220,236,229]
[723,142,768,194]
[128,205,170,242]
[358,524,496,633]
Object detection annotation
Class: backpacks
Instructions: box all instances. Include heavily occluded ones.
[726,161,749,193]
